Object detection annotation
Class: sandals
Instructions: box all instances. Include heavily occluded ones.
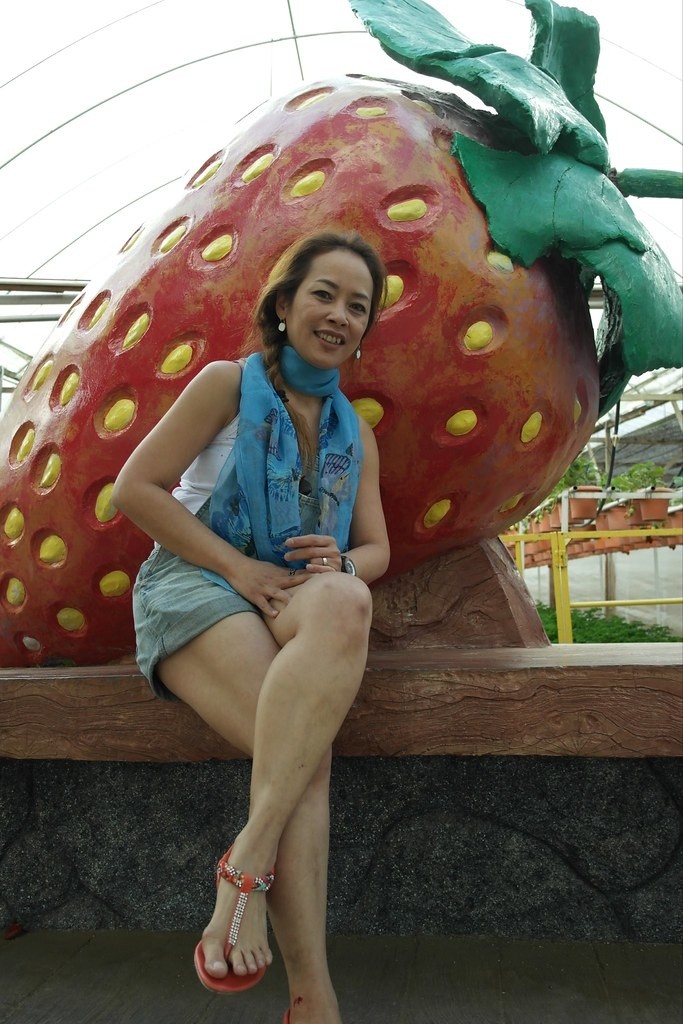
[194,845,276,996]
[284,1008,291,1024]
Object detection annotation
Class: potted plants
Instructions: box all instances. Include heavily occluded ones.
[500,456,683,568]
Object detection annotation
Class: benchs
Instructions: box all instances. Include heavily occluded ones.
[0,642,683,758]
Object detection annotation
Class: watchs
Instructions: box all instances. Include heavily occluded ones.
[340,556,357,578]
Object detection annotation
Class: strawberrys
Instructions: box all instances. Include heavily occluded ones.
[0,0,683,668]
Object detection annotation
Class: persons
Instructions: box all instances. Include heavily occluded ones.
[109,230,391,1023]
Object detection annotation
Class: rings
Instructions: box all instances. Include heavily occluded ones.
[288,568,298,576]
[322,557,328,566]
